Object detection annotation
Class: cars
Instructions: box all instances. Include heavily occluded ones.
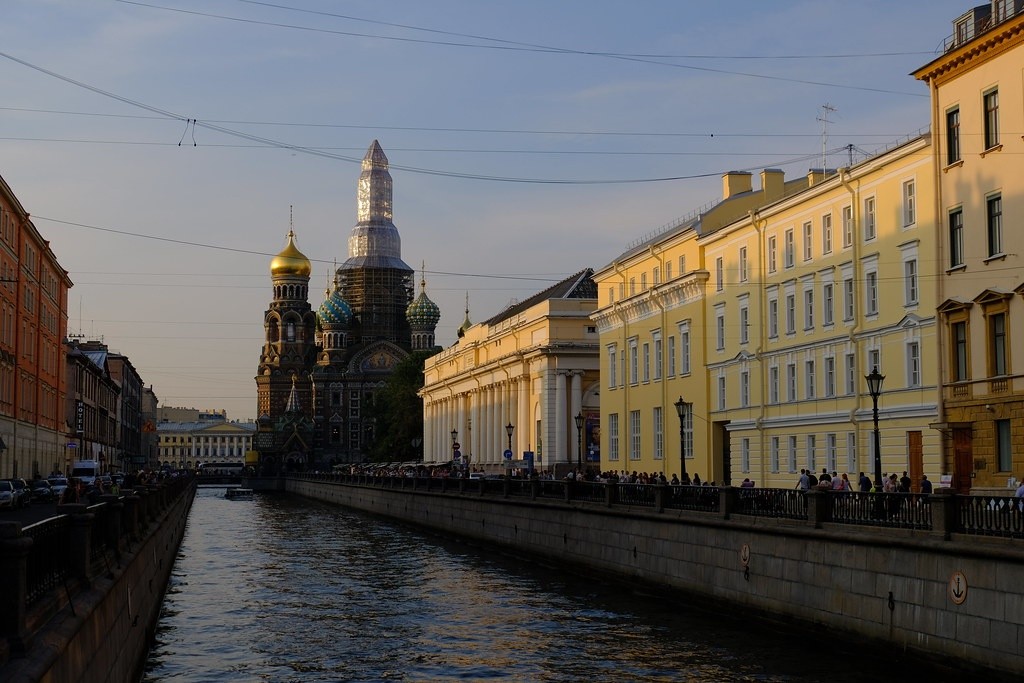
[100,472,128,491]
[0,480,18,507]
[31,480,53,501]
[11,478,31,506]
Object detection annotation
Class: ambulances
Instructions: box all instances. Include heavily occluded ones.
[70,459,101,495]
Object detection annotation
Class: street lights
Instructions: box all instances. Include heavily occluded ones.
[673,395,688,482]
[864,366,887,491]
[451,428,458,459]
[505,421,515,460]
[573,411,585,466]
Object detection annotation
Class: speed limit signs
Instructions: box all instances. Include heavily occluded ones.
[453,443,460,449]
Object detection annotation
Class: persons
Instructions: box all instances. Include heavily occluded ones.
[109,477,119,496]
[59,488,73,504]
[858,472,870,491]
[136,464,725,492]
[920,475,932,513]
[900,471,912,492]
[831,471,841,489]
[886,474,900,493]
[886,476,896,493]
[819,468,831,484]
[841,473,853,491]
[1015,477,1024,513]
[794,468,810,489]
[86,478,105,505]
[882,472,889,492]
[806,470,818,488]
[740,478,753,487]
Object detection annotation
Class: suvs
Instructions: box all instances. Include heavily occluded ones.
[48,477,70,502]
